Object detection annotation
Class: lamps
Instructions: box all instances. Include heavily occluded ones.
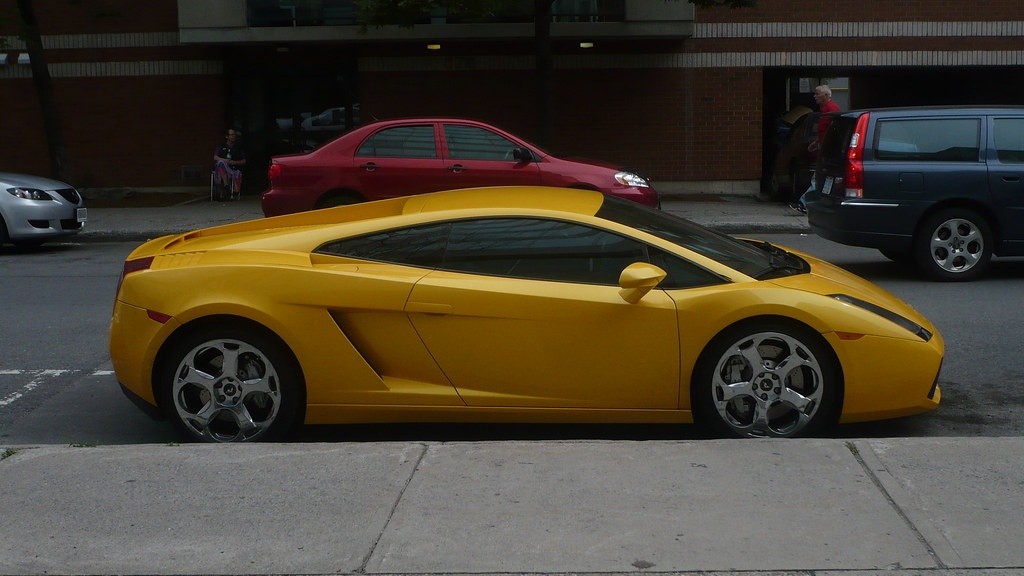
[0,54,9,65]
[277,47,288,51]
[426,44,440,49]
[580,42,594,48]
[17,54,30,64]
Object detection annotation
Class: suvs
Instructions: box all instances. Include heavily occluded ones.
[799,103,1024,283]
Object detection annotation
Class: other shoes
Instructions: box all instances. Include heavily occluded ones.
[790,202,807,216]
[224,184,231,191]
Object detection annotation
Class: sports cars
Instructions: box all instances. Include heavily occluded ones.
[105,183,948,441]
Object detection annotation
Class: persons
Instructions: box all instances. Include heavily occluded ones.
[788,84,840,215]
[214,128,246,201]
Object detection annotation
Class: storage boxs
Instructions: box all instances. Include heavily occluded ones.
[776,127,792,148]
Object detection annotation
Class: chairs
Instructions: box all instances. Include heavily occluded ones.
[211,153,241,202]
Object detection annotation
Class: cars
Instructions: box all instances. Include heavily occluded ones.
[274,104,379,134]
[261,116,661,218]
[0,170,88,250]
